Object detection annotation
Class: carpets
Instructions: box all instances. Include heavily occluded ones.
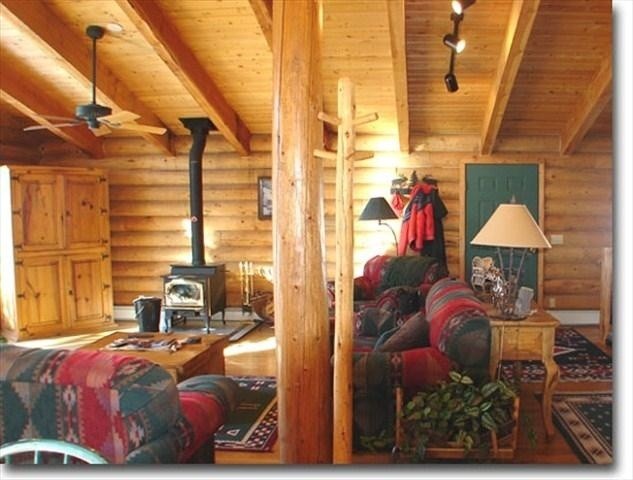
[196,375,278,453]
[533,391,612,464]
[502,326,612,384]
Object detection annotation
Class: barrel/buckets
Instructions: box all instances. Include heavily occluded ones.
[134,297,162,332]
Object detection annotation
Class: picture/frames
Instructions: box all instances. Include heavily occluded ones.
[258,176,272,220]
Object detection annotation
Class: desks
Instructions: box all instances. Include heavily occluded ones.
[487,300,561,442]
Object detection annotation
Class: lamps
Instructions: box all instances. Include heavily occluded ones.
[358,198,399,255]
[471,203,552,320]
[443,0,475,93]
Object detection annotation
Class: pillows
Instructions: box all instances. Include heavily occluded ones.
[373,312,430,352]
[359,308,394,337]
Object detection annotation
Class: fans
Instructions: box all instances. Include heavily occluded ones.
[23,26,167,138]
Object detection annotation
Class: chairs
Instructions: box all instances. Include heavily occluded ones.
[328,255,448,338]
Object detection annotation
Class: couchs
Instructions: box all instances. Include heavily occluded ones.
[0,344,247,465]
[331,278,491,448]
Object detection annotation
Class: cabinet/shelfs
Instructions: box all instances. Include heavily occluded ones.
[0,164,114,342]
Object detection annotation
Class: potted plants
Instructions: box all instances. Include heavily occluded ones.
[359,369,536,464]
